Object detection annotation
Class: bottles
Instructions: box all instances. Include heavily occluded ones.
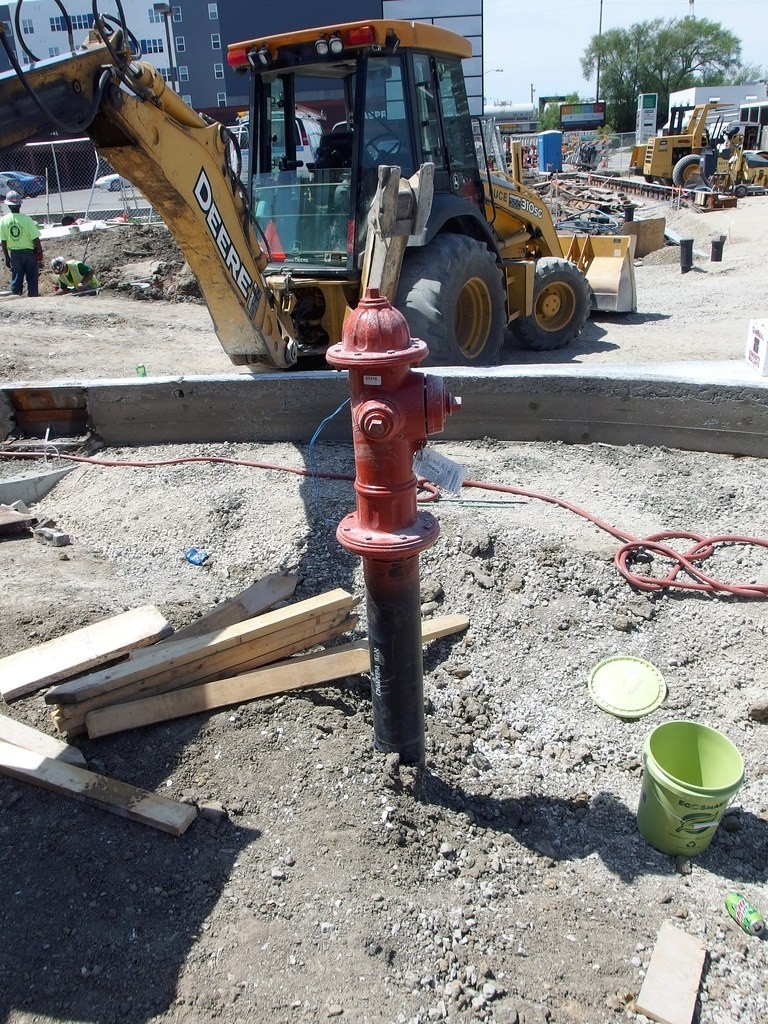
[136,364,146,377]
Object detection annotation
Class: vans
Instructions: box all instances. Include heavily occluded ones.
[234,117,323,184]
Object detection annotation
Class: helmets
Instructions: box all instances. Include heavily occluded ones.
[4,190,23,205]
[51,257,65,274]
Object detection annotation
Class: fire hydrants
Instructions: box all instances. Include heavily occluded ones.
[323,288,465,759]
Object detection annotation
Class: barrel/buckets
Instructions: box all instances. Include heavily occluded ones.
[636,720,745,857]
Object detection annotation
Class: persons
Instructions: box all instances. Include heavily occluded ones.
[51,257,101,297]
[309,129,378,218]
[0,190,45,298]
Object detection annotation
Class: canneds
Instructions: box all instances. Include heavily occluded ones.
[136,364,146,377]
[184,547,208,565]
[724,893,766,936]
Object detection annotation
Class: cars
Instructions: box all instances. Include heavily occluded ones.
[0,171,46,201]
[94,172,135,192]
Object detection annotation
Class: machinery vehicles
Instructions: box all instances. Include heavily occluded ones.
[0,0,639,366]
[629,98,768,196]
